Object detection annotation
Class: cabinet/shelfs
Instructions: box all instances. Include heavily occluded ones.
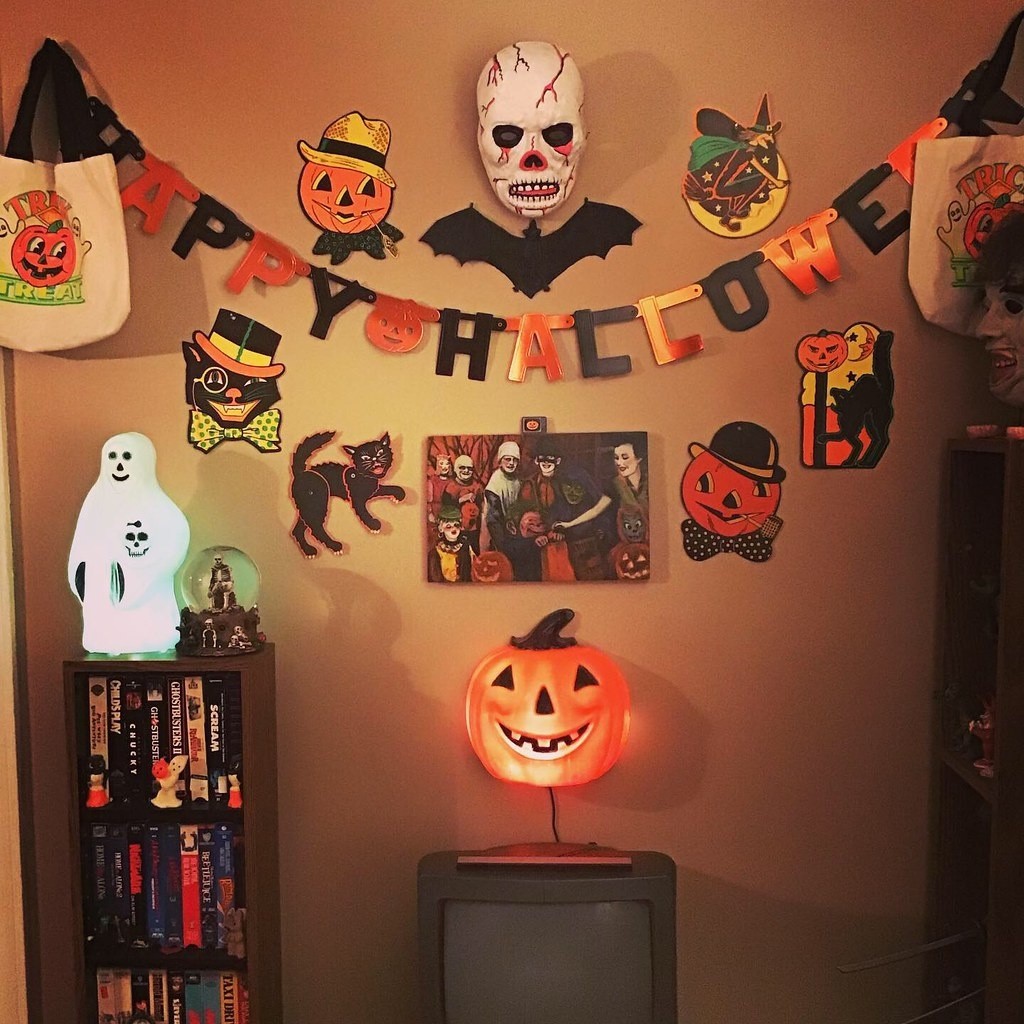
[60,645,283,1024]
[919,437,1024,1024]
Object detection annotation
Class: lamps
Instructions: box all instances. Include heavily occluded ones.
[455,607,634,864]
[67,432,189,656]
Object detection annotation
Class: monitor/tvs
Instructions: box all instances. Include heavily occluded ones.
[418,842,678,1024]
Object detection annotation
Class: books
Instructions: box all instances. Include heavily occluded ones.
[84,818,245,950]
[82,673,240,803]
[94,970,249,1024]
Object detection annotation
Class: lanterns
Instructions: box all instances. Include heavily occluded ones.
[466,609,631,788]
